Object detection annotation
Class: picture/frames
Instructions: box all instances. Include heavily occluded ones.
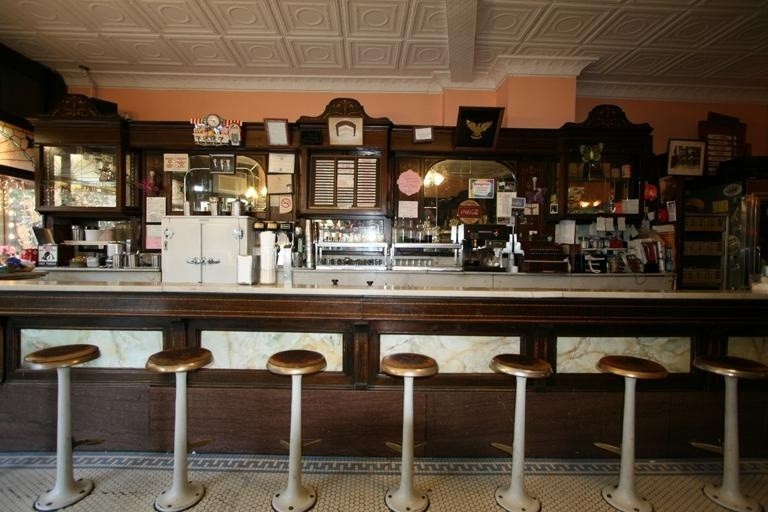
[663,136,708,178]
[208,153,235,175]
[263,117,291,147]
[450,104,505,154]
[411,124,435,144]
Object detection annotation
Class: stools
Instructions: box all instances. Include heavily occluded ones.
[488,352,560,512]
[264,348,327,512]
[380,352,439,511]
[144,347,216,511]
[595,353,668,511]
[688,354,766,511]
[20,343,100,511]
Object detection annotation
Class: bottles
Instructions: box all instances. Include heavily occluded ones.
[183,201,192,216]
[231,200,241,216]
[391,216,433,244]
[209,202,219,216]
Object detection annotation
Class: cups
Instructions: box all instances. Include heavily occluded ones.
[111,254,122,268]
[322,258,434,267]
[70,225,84,240]
[84,226,98,242]
[129,254,139,267]
[320,228,385,243]
[151,255,161,268]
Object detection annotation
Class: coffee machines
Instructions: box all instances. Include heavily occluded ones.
[252,220,296,258]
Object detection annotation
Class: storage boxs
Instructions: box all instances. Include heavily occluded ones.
[30,226,68,267]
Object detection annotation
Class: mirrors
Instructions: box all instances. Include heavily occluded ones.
[421,157,520,234]
[169,151,268,213]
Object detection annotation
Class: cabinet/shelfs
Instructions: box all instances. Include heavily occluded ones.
[160,215,258,286]
[551,103,654,231]
[313,240,388,271]
[387,240,463,273]
[32,142,142,219]
